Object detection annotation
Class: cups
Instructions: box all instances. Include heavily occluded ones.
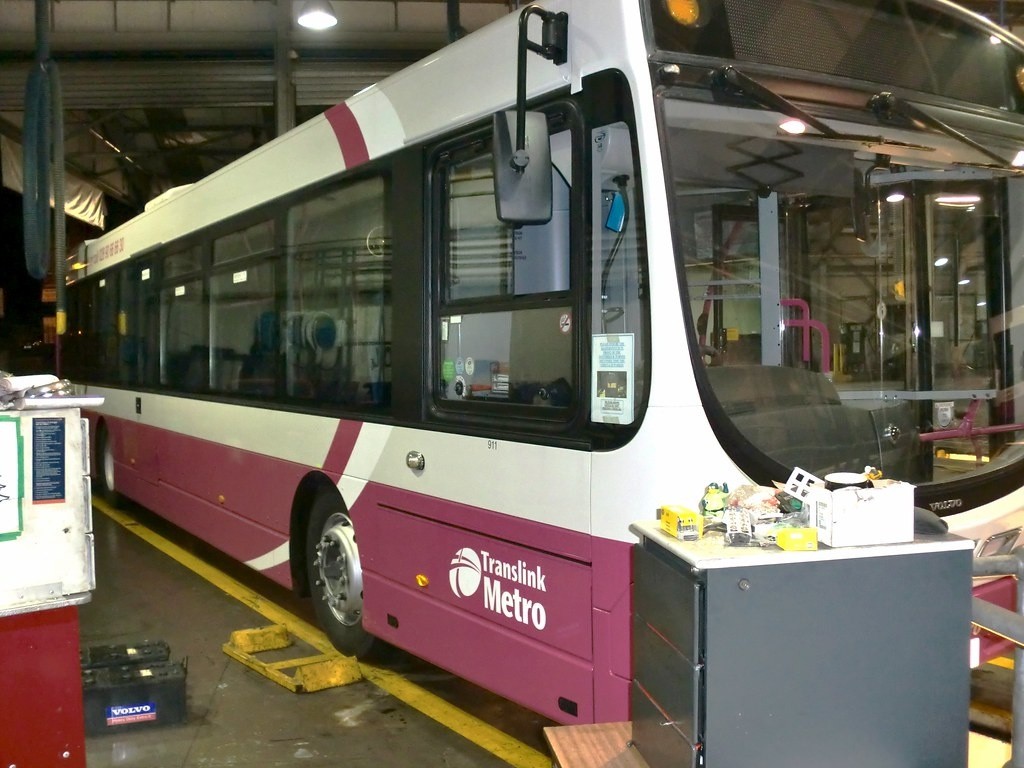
[824,472,874,491]
[726,504,750,544]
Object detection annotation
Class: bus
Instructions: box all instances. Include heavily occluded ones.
[68,0,1024,768]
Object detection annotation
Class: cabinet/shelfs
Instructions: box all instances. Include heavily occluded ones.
[624,522,973,768]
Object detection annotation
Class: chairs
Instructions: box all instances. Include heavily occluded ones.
[174,346,248,391]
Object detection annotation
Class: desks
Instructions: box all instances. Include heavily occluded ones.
[544,680,1012,767]
